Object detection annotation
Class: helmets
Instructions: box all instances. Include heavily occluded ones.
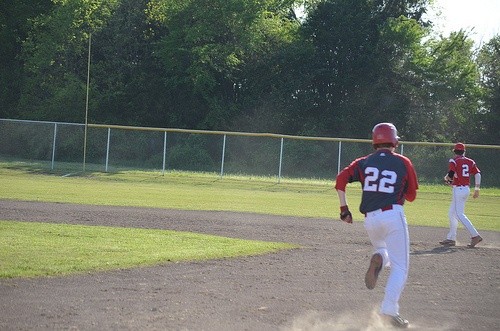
[372,123,398,144]
[455,143,464,151]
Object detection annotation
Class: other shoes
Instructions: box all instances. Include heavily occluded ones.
[365,253,383,290]
[380,313,409,328]
[439,239,456,246]
[468,235,483,248]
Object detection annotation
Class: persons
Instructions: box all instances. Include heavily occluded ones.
[439,143,484,248]
[335,123,419,328]
